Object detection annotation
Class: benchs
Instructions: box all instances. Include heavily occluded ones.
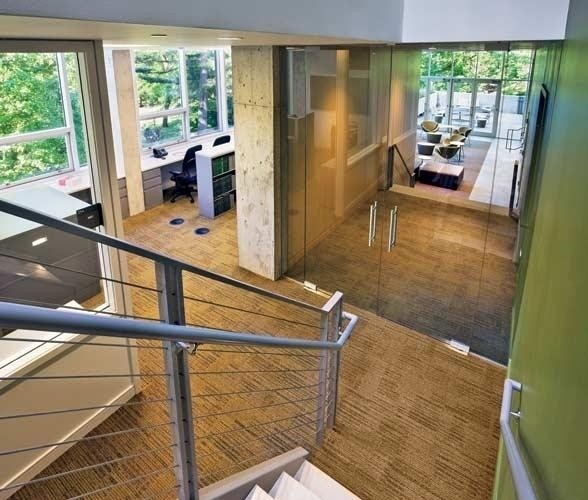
[418,160,464,191]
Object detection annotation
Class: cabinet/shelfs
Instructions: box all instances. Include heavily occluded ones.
[194,144,237,219]
[45,167,128,221]
[140,130,234,211]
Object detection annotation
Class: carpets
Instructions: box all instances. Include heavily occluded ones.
[413,133,491,200]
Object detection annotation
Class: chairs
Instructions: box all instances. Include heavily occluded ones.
[168,142,202,205]
[212,134,231,146]
[417,101,492,163]
